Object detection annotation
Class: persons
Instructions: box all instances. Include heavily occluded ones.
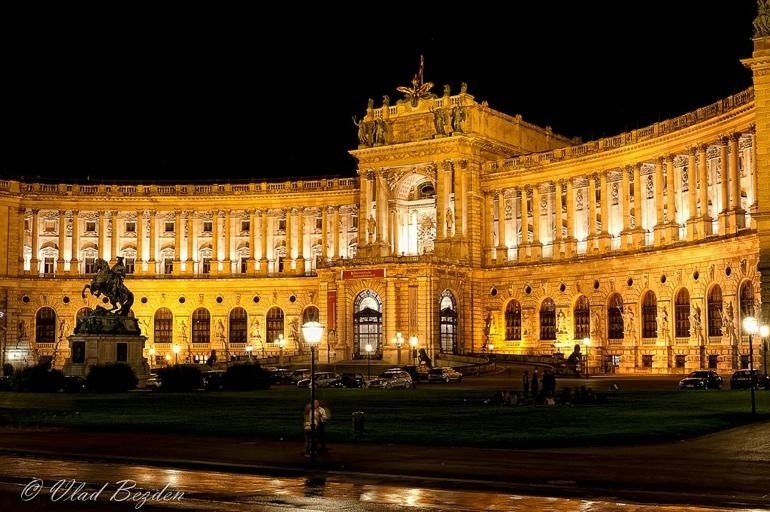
[450,104,464,132]
[428,104,446,137]
[374,116,389,144]
[311,398,330,454]
[350,113,371,146]
[492,368,598,407]
[185,357,189,364]
[109,255,127,300]
[303,399,315,458]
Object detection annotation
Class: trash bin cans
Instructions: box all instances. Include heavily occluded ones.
[353,411,364,431]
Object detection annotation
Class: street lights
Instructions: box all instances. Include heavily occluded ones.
[410,338,416,364]
[245,345,254,358]
[273,333,285,366]
[741,315,770,413]
[582,338,593,379]
[392,333,404,364]
[298,321,325,468]
[148,347,180,364]
[364,345,373,379]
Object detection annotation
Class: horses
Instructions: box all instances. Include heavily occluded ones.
[81,258,134,316]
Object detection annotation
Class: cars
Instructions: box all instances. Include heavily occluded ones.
[428,366,463,382]
[678,369,724,391]
[729,366,770,390]
[145,365,412,390]
